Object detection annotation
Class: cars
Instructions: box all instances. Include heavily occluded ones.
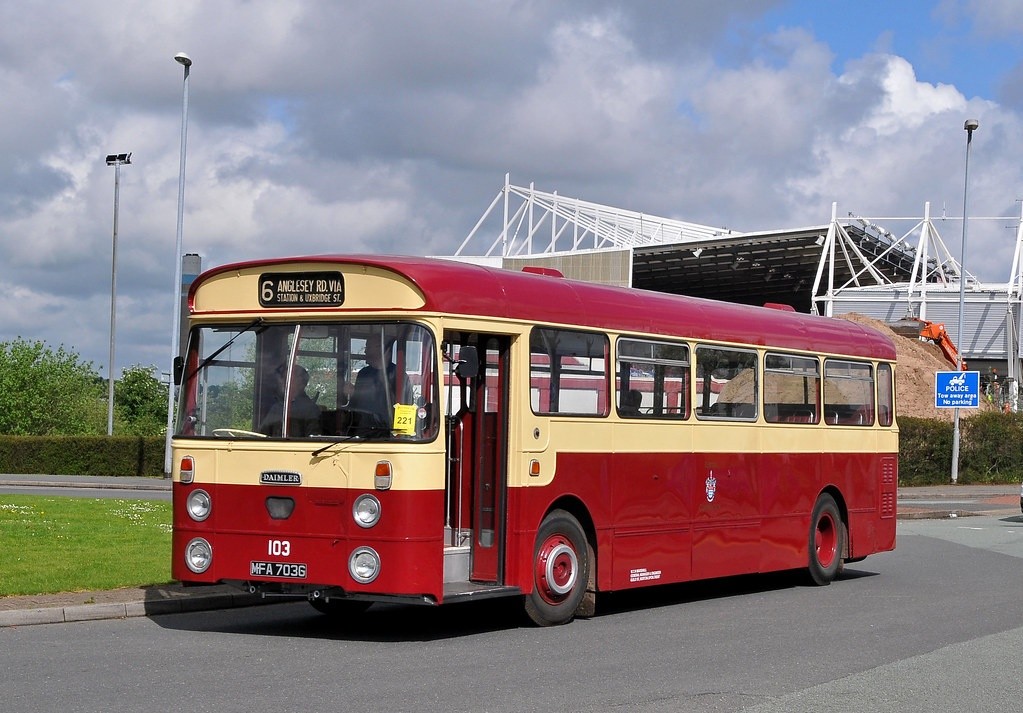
[980,373,1023,397]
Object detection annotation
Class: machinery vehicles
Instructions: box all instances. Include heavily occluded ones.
[890,315,969,371]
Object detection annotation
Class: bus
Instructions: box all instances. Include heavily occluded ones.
[140,253,902,628]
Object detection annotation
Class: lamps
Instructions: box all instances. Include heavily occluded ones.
[691,248,705,258]
[729,261,742,272]
[762,273,773,283]
[792,284,801,291]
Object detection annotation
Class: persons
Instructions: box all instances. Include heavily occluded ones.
[257,364,324,437]
[342,336,415,436]
[620,390,643,420]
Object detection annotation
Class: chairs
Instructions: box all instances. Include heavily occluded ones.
[642,402,887,426]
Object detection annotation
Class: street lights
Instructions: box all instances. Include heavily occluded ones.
[105,151,135,436]
[949,118,981,484]
[163,52,194,480]
